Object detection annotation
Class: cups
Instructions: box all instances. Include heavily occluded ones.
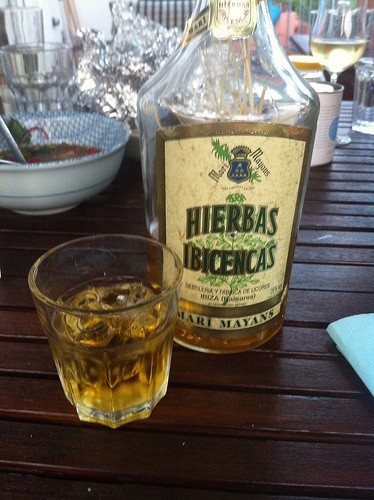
[306,81,345,166]
[1,7,44,49]
[288,0,325,80]
[352,57,374,135]
[27,234,184,429]
[0,42,75,112]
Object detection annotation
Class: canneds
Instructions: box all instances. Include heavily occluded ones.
[306,82,344,167]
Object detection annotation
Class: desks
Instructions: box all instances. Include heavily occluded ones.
[1,99,374,500]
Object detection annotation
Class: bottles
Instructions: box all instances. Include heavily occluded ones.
[138,0,321,355]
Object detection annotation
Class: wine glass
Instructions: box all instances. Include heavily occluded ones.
[311,0,368,145]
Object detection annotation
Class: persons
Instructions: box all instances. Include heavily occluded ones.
[202,0,308,56]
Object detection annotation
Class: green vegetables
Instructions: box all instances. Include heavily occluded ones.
[0,118,89,163]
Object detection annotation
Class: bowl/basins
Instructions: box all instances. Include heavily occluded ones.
[1,110,129,215]
